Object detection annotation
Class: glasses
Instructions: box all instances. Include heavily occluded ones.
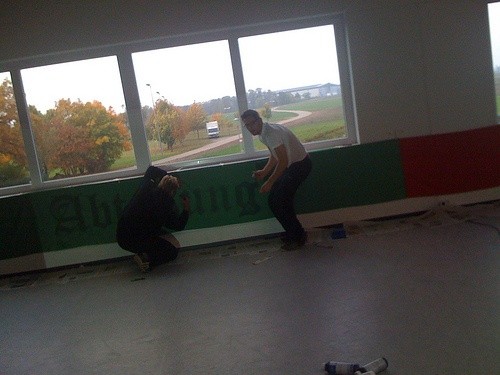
[244,119,259,128]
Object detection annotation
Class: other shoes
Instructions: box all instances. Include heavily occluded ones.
[281,230,308,252]
[134,253,150,272]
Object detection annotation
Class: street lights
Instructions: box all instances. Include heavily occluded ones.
[146,83,165,152]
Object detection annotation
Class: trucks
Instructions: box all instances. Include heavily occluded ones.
[205,121,220,139]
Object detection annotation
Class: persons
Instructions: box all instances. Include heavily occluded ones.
[117,167,190,272]
[241,110,311,251]
